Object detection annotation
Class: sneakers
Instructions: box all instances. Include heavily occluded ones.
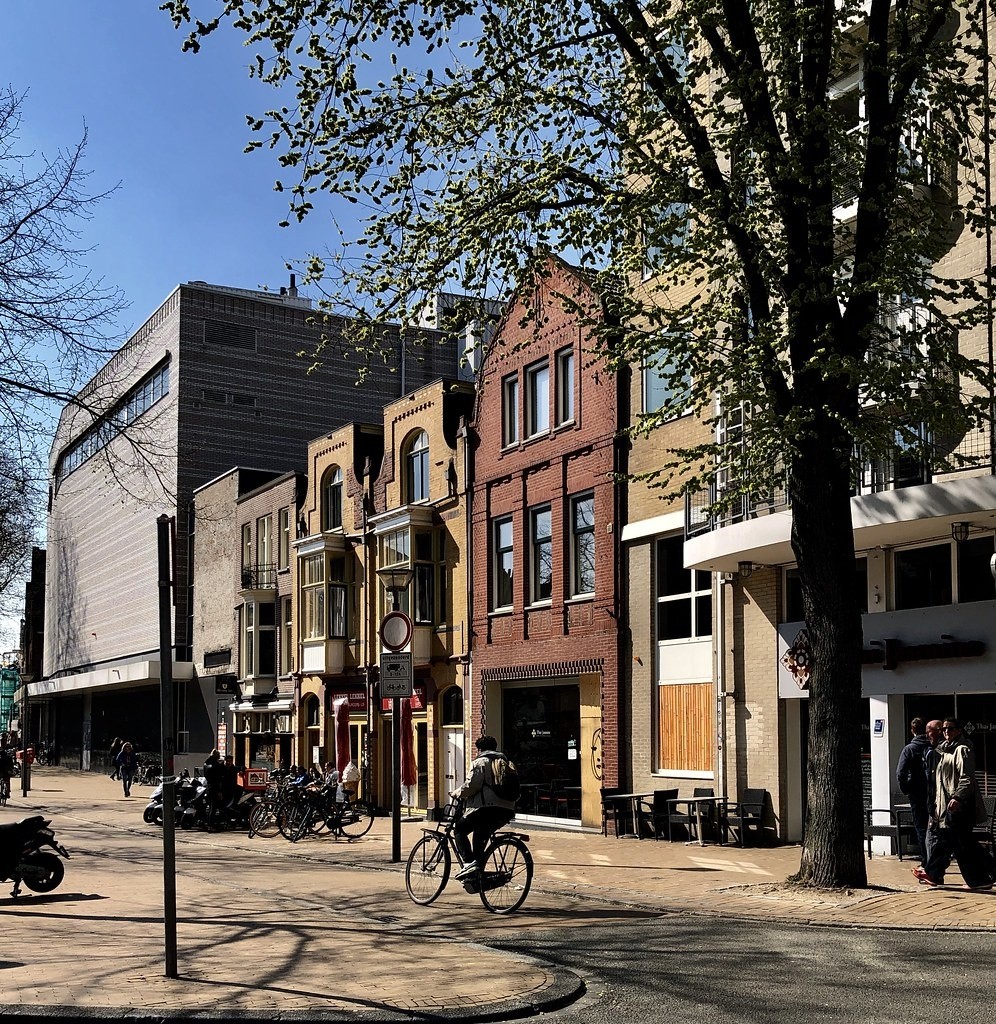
[963,879,993,890]
[911,868,937,886]
[465,871,482,882]
[455,860,480,880]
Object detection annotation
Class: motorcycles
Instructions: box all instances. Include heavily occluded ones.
[0,815,72,901]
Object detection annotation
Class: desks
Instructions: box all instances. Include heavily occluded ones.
[520,784,551,814]
[666,797,729,847]
[895,804,911,808]
[605,793,654,840]
[565,786,581,818]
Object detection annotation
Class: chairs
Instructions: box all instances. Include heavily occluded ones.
[972,797,996,862]
[536,778,580,818]
[599,785,766,849]
[866,809,917,862]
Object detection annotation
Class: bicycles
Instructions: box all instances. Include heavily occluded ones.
[404,791,534,916]
[134,751,376,844]
[0,778,8,807]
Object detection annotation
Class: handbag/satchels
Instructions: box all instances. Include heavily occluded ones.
[945,781,987,827]
[133,773,139,783]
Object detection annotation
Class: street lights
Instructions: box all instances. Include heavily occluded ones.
[19,672,34,797]
[376,566,415,856]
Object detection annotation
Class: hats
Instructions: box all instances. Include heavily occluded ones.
[224,755,233,760]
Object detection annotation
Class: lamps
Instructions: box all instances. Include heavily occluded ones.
[951,521,970,543]
[737,561,753,577]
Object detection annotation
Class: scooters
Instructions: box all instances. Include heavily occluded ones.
[6,742,49,778]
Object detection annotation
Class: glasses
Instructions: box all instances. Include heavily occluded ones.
[941,726,960,732]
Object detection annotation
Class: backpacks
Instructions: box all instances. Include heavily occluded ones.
[110,746,116,756]
[485,754,521,801]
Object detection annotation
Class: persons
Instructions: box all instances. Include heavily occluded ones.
[110,737,138,797]
[203,748,247,832]
[0,751,14,798]
[285,761,340,804]
[896,717,996,892]
[451,736,516,881]
